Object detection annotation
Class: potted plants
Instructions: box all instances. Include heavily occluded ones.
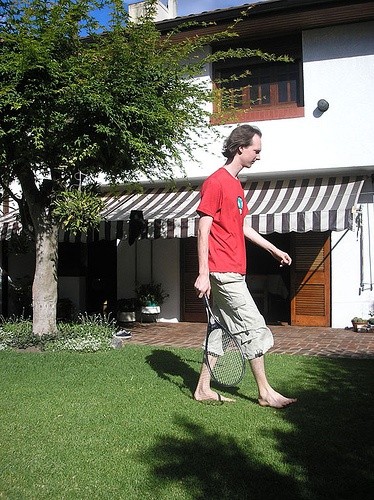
[136,281,169,314]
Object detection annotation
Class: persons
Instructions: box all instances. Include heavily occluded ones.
[194,125,297,409]
